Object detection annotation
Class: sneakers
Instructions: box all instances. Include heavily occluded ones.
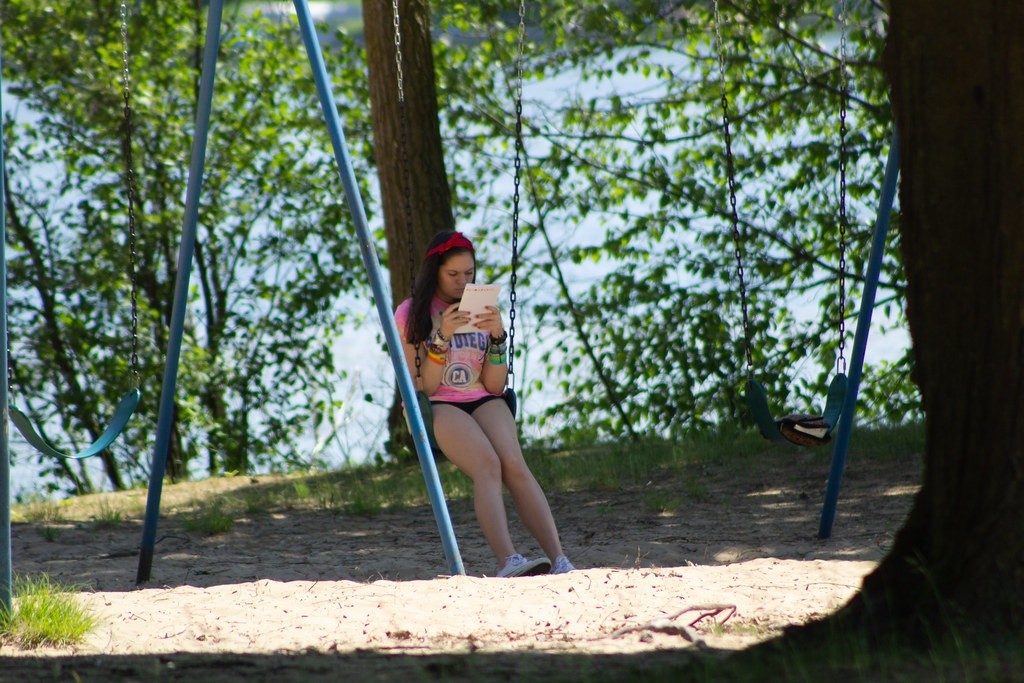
[552,554,574,574]
[495,554,551,578]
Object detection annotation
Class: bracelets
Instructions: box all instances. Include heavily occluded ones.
[428,327,451,365]
[489,329,508,364]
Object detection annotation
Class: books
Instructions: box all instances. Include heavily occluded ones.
[454,283,501,333]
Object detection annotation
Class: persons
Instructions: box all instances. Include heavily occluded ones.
[389,230,576,579]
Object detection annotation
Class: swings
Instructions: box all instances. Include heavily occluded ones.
[392,1,525,449]
[708,3,857,446]
[4,1,144,461]
